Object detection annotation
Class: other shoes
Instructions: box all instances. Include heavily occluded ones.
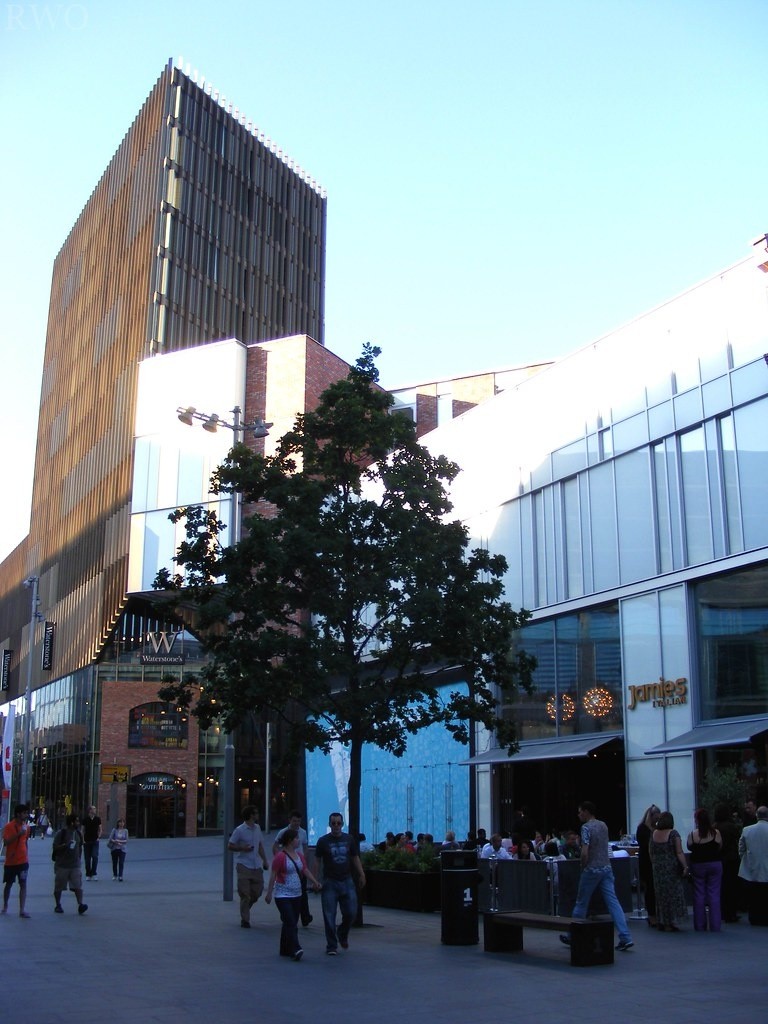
[55,906,64,913]
[559,934,570,945]
[118,876,123,881]
[3,904,9,914]
[302,916,312,926]
[94,875,98,880]
[660,923,679,932]
[112,875,117,880]
[86,875,90,880]
[615,940,634,950]
[241,921,250,927]
[20,912,31,918]
[328,946,337,955]
[78,904,88,913]
[291,949,304,961]
[338,933,348,948]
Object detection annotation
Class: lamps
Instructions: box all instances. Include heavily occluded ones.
[545,693,577,721]
[582,687,616,721]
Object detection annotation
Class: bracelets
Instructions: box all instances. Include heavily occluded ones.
[98,836,101,839]
[683,866,688,869]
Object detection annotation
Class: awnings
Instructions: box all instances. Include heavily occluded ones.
[643,719,768,755]
[460,737,617,767]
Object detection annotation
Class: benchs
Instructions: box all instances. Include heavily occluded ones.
[482,908,617,969]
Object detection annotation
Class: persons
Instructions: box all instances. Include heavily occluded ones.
[635,804,662,927]
[648,811,689,931]
[227,806,269,928]
[687,809,723,932]
[356,828,636,918]
[27,810,39,841]
[559,801,634,951]
[713,798,768,925]
[80,805,102,880]
[272,811,313,926]
[108,817,128,881]
[0,804,32,918]
[313,812,366,955]
[53,815,89,914]
[38,807,51,840]
[265,829,322,960]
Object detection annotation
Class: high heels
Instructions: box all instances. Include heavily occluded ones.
[647,918,658,928]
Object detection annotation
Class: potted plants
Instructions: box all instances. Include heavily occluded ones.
[361,840,440,913]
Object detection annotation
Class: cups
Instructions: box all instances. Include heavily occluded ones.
[70,840,77,849]
[22,825,27,831]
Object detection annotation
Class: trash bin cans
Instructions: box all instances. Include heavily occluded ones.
[440,849,480,945]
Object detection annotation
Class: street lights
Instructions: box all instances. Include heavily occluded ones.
[181,404,274,896]
[18,577,46,802]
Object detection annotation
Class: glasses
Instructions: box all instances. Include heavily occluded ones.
[331,822,342,826]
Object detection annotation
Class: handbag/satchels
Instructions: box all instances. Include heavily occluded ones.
[108,828,115,848]
[672,856,684,872]
